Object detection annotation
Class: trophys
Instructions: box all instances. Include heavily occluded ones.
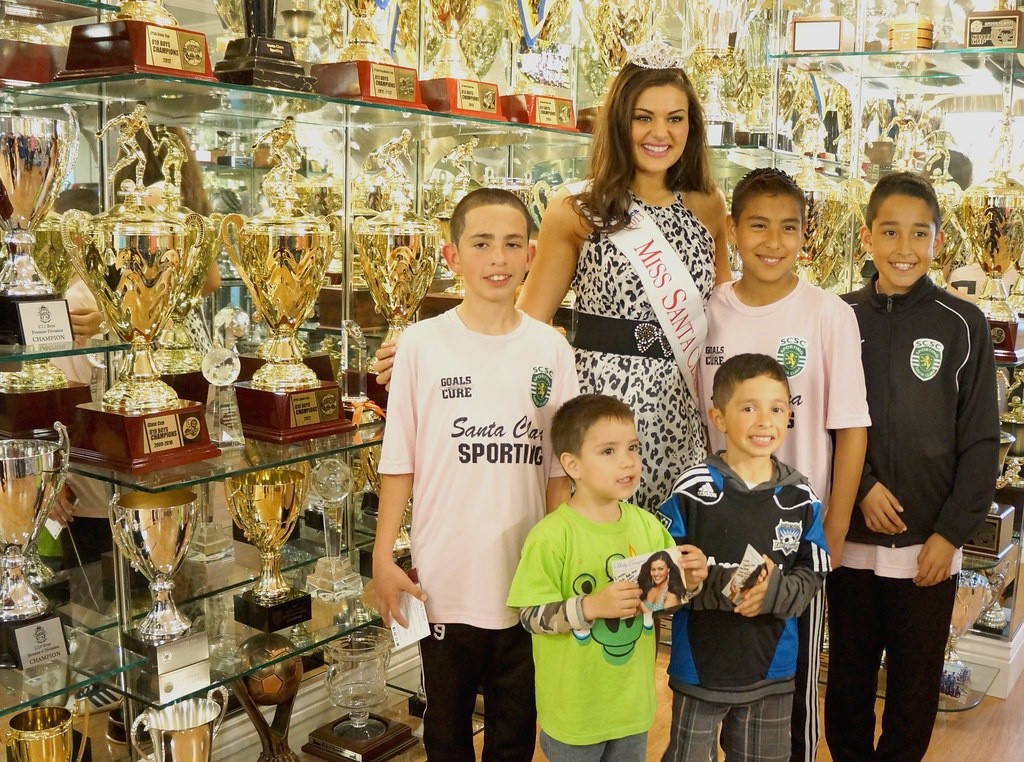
[861,96,1024,321]
[1,0,852,169]
[88,201,443,411]
[0,419,392,761]
[939,573,1006,702]
[0,102,79,295]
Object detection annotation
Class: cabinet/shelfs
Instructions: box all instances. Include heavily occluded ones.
[0,0,1024,762]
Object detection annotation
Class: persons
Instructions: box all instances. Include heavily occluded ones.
[696,165,999,762]
[654,352,831,762]
[633,551,687,618]
[373,186,581,762]
[374,30,733,662]
[727,561,768,606]
[506,394,707,762]
[69,123,221,340]
